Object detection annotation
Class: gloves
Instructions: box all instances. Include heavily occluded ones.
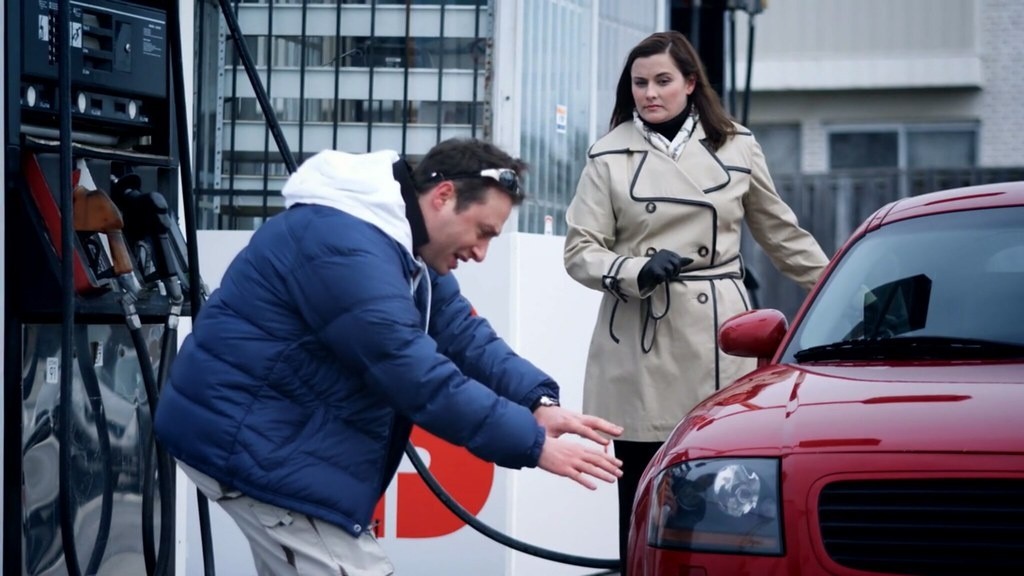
[642,249,694,283]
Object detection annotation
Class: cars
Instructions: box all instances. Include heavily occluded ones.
[625,180,1024,576]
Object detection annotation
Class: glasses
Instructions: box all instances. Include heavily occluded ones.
[430,167,520,197]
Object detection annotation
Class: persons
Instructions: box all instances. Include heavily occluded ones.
[563,30,876,576]
[151,137,624,575]
